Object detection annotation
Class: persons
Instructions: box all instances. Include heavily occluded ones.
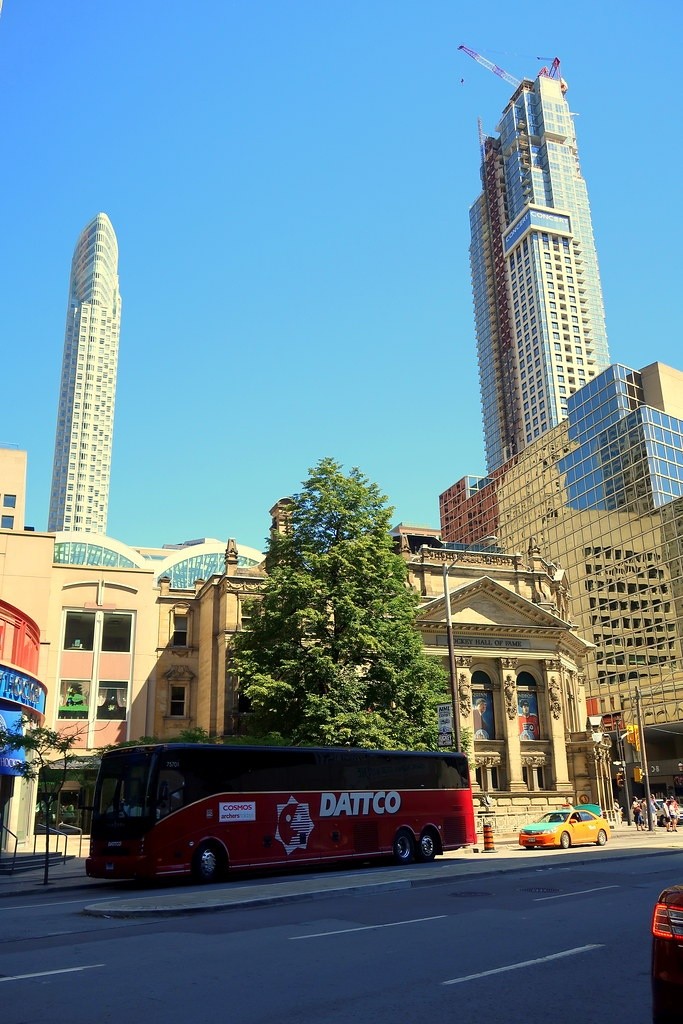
[615,799,619,809]
[631,793,679,833]
[473,698,487,735]
[519,699,539,740]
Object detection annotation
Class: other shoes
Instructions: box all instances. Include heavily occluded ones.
[667,829,672,832]
[672,829,677,832]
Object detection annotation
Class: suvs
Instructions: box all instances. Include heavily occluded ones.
[630,799,683,827]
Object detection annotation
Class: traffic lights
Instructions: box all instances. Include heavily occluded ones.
[616,773,625,787]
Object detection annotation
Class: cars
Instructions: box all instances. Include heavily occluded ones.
[651,885,683,982]
[518,804,612,850]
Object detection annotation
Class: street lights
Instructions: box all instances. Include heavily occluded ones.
[635,669,683,832]
[441,535,499,753]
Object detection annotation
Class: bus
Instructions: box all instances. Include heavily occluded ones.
[77,742,478,885]
